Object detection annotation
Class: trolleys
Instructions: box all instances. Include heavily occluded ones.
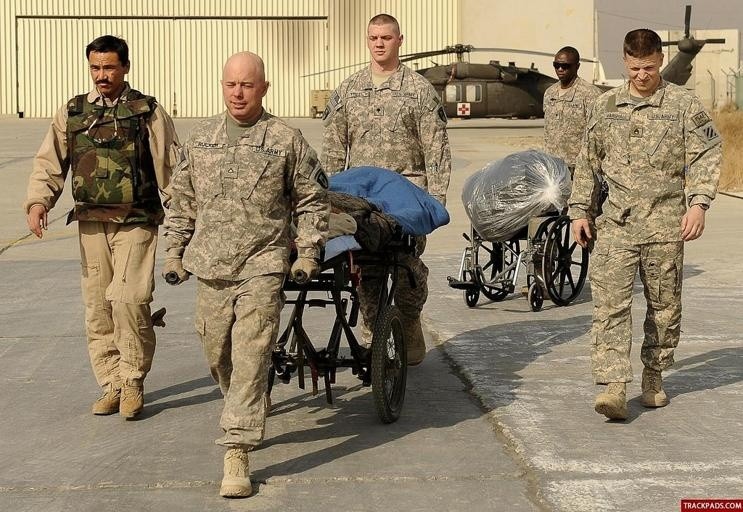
[164,193,417,424]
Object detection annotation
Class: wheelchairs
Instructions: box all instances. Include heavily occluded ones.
[447,162,592,311]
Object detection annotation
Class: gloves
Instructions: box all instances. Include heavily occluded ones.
[162,255,189,286]
[290,257,318,285]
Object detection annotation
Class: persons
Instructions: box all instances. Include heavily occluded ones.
[24,35,182,419]
[320,14,451,366]
[521,46,605,299]
[162,51,330,498]
[566,29,724,421]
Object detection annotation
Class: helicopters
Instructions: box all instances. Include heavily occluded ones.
[293,2,734,123]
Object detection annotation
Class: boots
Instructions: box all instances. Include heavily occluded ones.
[219,445,253,499]
[404,317,426,366]
[641,368,667,407]
[120,383,143,418]
[93,390,120,415]
[595,382,628,421]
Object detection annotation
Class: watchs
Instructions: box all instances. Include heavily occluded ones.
[698,203,710,210]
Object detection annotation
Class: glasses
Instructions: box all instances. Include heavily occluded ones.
[553,61,571,71]
